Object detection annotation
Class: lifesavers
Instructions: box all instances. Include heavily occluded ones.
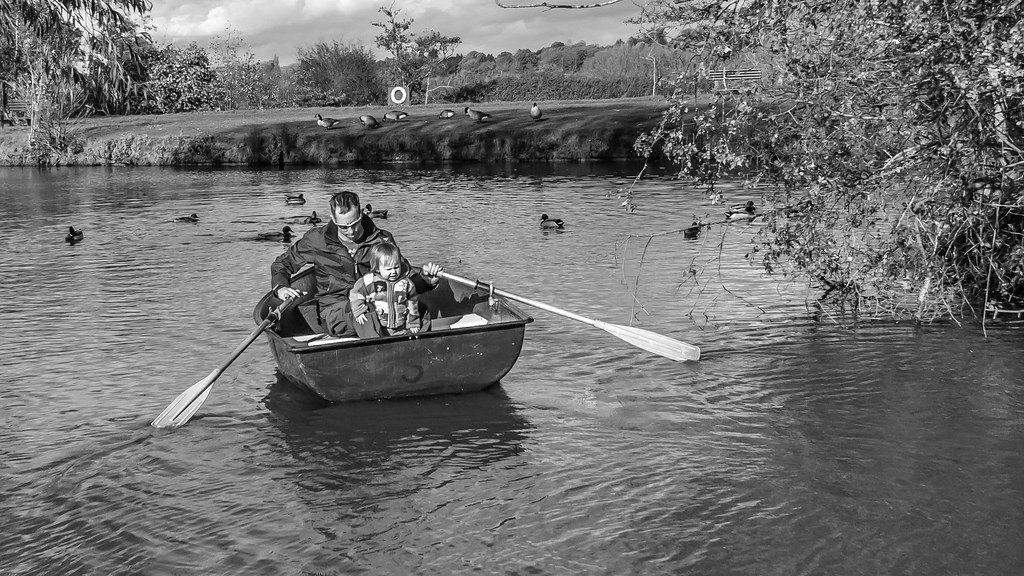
[391,87,406,104]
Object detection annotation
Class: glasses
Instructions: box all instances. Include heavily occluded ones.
[329,213,363,230]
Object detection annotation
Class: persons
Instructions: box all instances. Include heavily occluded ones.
[271,189,443,338]
[348,240,421,335]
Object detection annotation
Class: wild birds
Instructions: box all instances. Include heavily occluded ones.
[304,210,322,224]
[313,113,340,131]
[359,114,380,130]
[285,194,306,206]
[530,102,542,122]
[439,109,455,119]
[464,107,492,124]
[173,213,199,222]
[683,221,701,239]
[539,213,565,230]
[256,225,295,243]
[364,203,388,220]
[65,225,84,245]
[723,200,756,222]
[382,110,411,123]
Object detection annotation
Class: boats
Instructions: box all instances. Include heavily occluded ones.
[252,262,540,403]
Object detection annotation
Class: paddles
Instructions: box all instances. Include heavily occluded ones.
[151,287,302,429]
[421,264,702,363]
